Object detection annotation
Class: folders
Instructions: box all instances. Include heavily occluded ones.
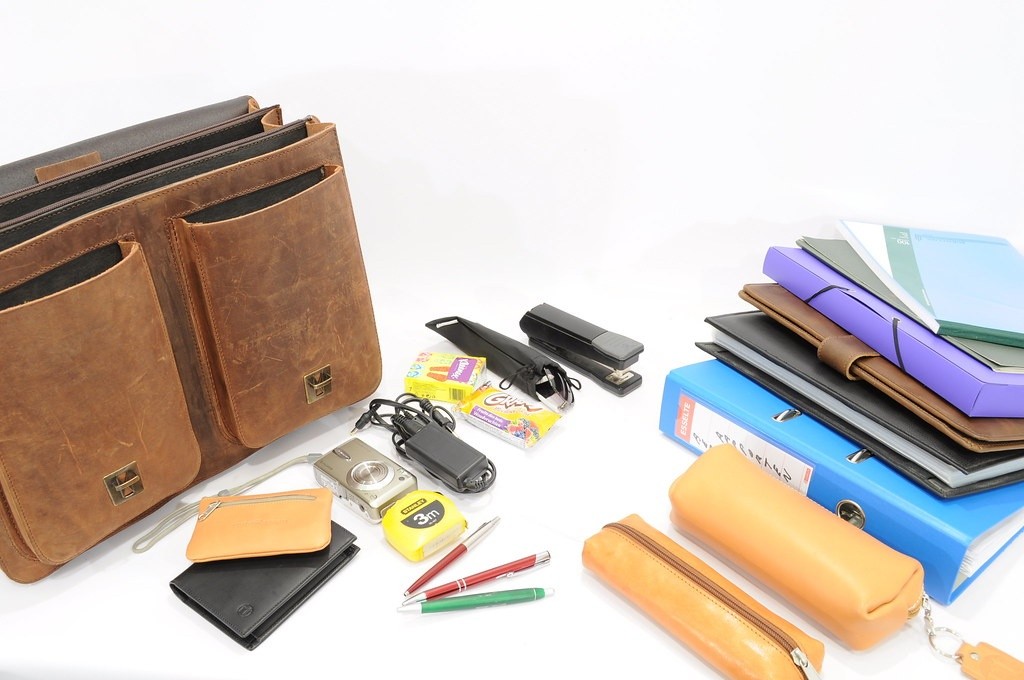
[656,359,1024,604]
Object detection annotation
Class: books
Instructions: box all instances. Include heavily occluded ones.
[660,218,1024,605]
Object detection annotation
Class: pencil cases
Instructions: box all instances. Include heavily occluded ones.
[666,443,928,651]
[582,513,827,680]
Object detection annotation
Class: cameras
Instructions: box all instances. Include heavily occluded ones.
[313,438,418,525]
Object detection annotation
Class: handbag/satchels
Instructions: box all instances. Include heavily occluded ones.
[2,95,385,584]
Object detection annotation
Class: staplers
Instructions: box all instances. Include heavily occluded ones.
[519,302,646,397]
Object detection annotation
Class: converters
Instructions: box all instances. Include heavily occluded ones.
[405,421,488,494]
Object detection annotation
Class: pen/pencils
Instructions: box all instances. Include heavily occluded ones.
[395,588,558,613]
[403,516,501,597]
[402,550,551,606]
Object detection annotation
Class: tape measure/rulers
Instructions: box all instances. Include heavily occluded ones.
[381,489,467,562]
